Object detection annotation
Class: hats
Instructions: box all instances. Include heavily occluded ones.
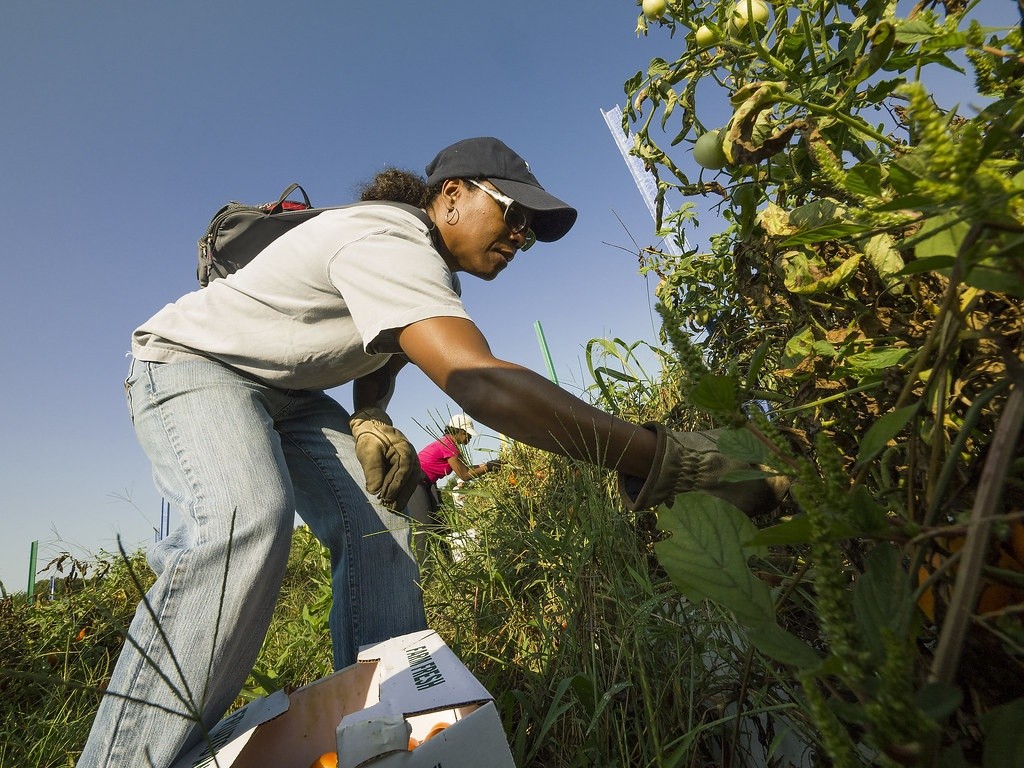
[425,136,578,243]
[449,415,477,436]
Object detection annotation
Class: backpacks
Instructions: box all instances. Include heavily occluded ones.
[196,183,441,289]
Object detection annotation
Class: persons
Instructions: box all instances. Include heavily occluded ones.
[71,138,790,768]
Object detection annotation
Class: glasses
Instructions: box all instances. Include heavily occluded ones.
[463,178,536,252]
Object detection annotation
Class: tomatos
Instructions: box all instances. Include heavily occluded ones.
[310,721,453,768]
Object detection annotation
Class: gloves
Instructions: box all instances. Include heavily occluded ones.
[483,460,502,474]
[616,420,792,513]
[348,406,421,514]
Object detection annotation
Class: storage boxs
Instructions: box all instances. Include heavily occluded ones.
[176,627,516,768]
[446,529,478,565]
